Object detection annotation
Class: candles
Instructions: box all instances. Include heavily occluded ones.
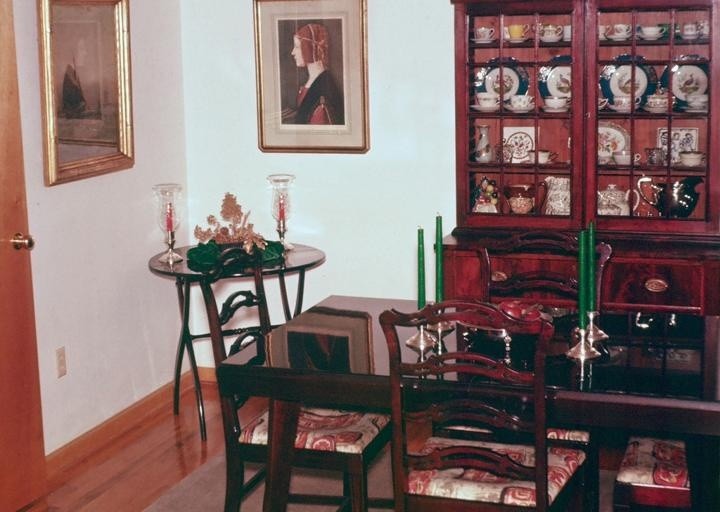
[436,213,444,303]
[278,193,284,221]
[417,225,425,330]
[166,202,172,231]
[579,221,595,329]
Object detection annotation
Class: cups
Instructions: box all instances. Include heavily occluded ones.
[605,95,709,114]
[527,150,549,164]
[475,92,500,106]
[613,151,641,165]
[544,97,567,108]
[501,181,547,215]
[472,23,703,42]
[510,95,532,108]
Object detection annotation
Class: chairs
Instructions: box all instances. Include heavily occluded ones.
[613,429,694,512]
[200,247,395,512]
[378,300,601,511]
[430,234,612,512]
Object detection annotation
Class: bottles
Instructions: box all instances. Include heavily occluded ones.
[645,148,664,168]
[152,184,184,262]
[266,174,296,251]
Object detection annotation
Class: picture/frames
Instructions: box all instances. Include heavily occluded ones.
[252,1,372,154]
[37,0,136,188]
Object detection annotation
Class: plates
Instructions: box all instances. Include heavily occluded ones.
[505,105,535,113]
[474,57,530,105]
[600,55,658,109]
[542,107,569,112]
[568,122,630,164]
[538,55,572,105]
[660,54,709,112]
[469,105,500,113]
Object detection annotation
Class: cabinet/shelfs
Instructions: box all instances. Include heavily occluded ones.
[432,0,718,317]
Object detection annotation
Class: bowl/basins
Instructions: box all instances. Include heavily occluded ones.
[679,151,703,166]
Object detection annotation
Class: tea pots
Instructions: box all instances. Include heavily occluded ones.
[597,183,640,215]
[636,176,704,217]
[543,176,570,215]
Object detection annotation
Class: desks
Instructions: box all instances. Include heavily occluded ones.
[215,295,715,512]
[146,244,326,440]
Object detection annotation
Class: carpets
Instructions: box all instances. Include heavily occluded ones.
[139,439,618,512]
